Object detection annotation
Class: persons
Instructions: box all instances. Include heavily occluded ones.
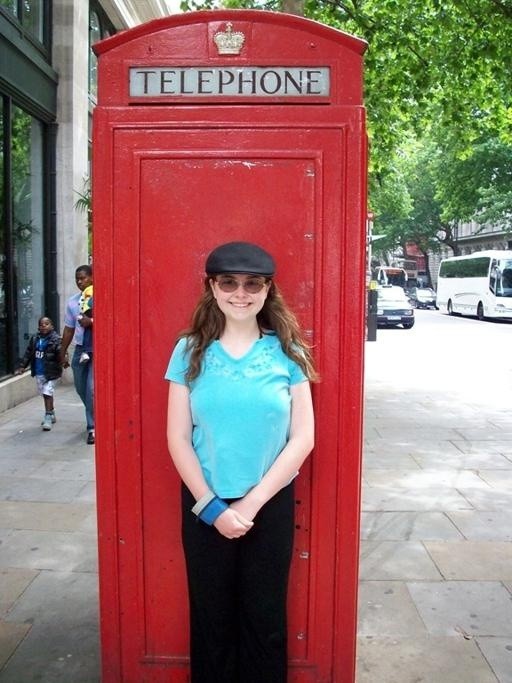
[161,241,319,682]
[57,264,96,444]
[79,285,94,362]
[17,316,70,430]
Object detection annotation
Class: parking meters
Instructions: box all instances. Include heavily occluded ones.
[368,290,378,341]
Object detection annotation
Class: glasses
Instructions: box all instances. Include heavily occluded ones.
[218,278,263,292]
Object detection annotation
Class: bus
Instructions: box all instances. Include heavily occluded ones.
[436,249,512,320]
[391,259,418,286]
[372,266,408,294]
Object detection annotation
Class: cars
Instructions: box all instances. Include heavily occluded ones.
[377,283,415,329]
[410,287,440,310]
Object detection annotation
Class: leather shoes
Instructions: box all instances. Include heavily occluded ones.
[87,432,95,443]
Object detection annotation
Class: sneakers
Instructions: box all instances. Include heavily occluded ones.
[41,413,55,429]
[78,353,89,365]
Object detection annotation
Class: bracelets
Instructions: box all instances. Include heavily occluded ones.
[91,317,94,323]
[190,490,229,526]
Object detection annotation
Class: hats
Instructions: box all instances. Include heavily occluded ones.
[205,241,275,276]
